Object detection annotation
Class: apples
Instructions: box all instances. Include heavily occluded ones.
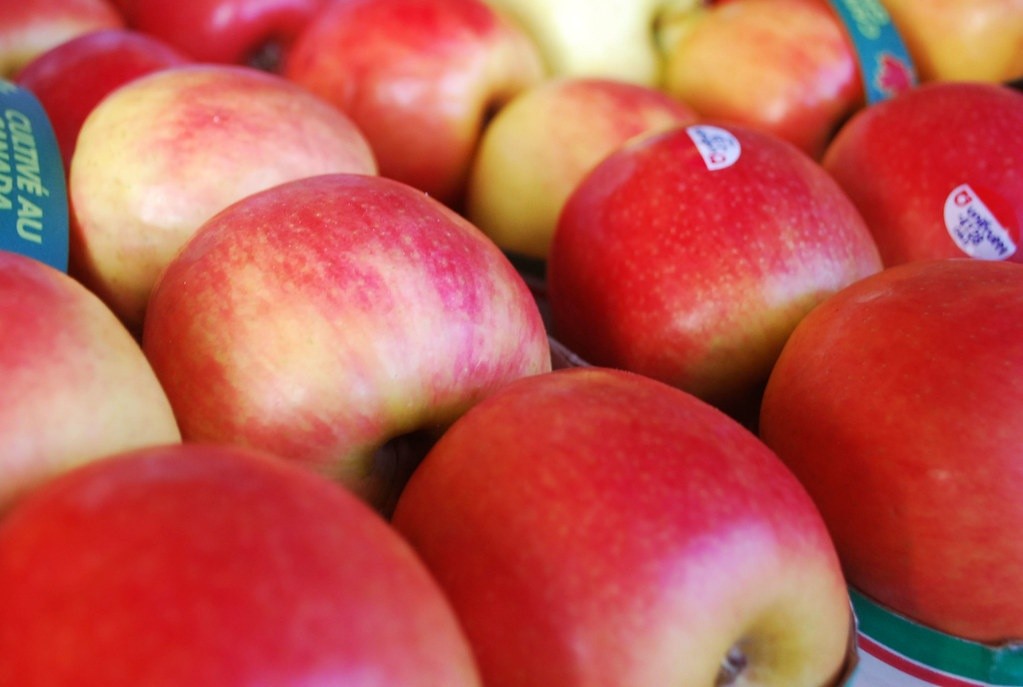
[0,0,1023,687]
